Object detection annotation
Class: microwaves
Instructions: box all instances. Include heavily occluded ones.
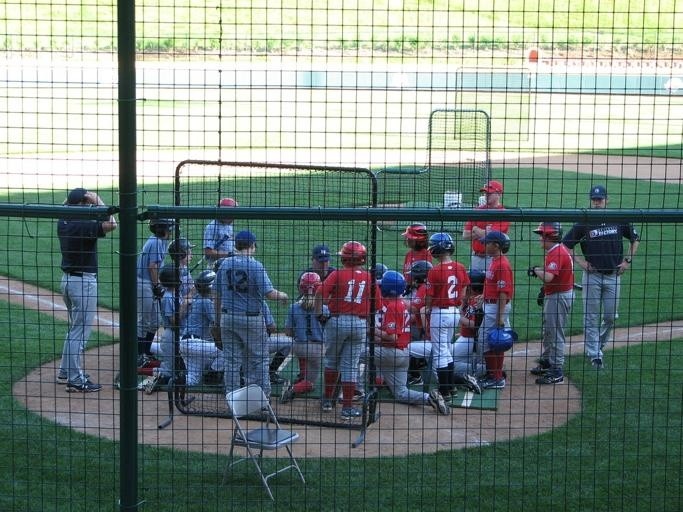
[189,234,229,273]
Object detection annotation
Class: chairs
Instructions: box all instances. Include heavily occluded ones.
[320,394,333,413]
[340,405,363,418]
[202,371,224,384]
[57,371,104,392]
[269,372,295,401]
[137,375,157,394]
[529,361,565,384]
[587,350,605,369]
[136,349,158,371]
[407,372,507,416]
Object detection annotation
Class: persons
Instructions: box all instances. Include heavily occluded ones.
[339,270,450,415]
[213,231,289,417]
[313,241,383,417]
[559,184,641,368]
[204,198,238,270]
[137,216,174,368]
[53,188,117,393]
[297,243,336,374]
[137,237,295,394]
[525,220,577,385]
[475,230,512,388]
[401,223,432,285]
[463,180,509,353]
[280,272,330,404]
[339,260,431,403]
[425,232,470,400]
[451,267,507,395]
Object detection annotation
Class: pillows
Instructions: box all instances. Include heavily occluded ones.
[217,198,239,219]
[300,272,322,290]
[534,220,563,241]
[148,215,180,238]
[400,222,511,294]
[488,328,517,351]
[335,241,368,265]
[368,262,406,298]
[149,238,216,292]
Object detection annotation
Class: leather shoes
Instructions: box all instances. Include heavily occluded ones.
[152,283,164,298]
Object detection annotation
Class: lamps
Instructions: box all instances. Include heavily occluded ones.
[222,382,308,501]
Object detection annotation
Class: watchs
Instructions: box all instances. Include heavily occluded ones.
[624,257,632,263]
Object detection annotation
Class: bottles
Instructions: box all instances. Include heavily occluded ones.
[235,229,259,247]
[312,244,334,262]
[67,188,90,204]
[480,180,503,195]
[590,184,608,199]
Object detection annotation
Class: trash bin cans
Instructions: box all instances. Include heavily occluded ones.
[624,257,632,263]
